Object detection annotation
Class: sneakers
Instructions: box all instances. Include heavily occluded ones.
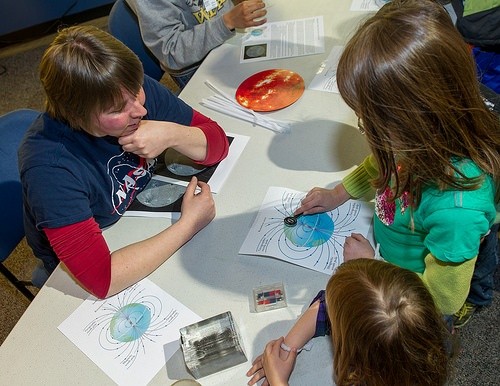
[452,300,478,328]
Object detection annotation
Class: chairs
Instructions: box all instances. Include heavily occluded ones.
[0,109,41,302]
[108,0,166,82]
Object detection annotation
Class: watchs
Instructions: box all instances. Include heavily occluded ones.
[280,342,302,356]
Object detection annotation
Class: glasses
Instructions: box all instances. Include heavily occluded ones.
[357,118,365,134]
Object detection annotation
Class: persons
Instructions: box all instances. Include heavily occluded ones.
[246,257,457,386]
[125,0,267,90]
[291,0,500,327]
[18,25,229,298]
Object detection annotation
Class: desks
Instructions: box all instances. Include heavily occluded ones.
[0,0,385,386]
